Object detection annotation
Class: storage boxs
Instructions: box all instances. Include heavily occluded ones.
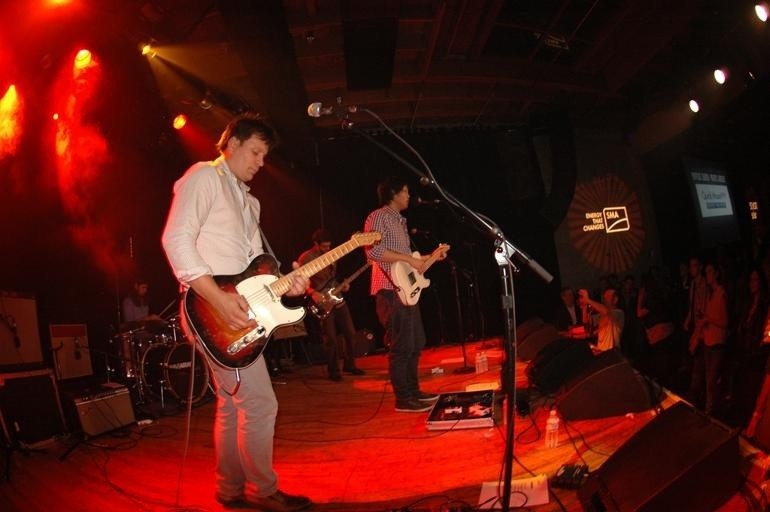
[68,382,138,439]
[48,324,93,381]
[0,292,43,369]
[0,369,70,454]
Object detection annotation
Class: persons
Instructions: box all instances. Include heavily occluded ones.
[686,259,711,396]
[582,289,624,353]
[737,271,763,380]
[123,281,160,324]
[363,167,446,412]
[556,288,587,337]
[298,230,364,381]
[162,110,310,512]
[691,264,728,416]
[611,276,649,369]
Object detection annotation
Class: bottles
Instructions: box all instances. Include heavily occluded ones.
[476,352,485,374]
[545,410,559,448]
[482,351,488,373]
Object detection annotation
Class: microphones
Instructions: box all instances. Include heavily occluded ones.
[306,102,358,118]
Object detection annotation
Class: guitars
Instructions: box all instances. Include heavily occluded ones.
[180,230,383,372]
[388,242,452,309]
[306,260,381,321]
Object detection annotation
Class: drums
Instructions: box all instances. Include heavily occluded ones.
[141,341,210,407]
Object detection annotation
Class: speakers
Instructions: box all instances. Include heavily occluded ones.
[575,400,741,512]
[557,358,656,421]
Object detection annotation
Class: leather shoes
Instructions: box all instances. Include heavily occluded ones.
[395,400,434,412]
[247,490,313,512]
[417,392,440,401]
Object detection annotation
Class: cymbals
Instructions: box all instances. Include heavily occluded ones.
[119,318,169,334]
